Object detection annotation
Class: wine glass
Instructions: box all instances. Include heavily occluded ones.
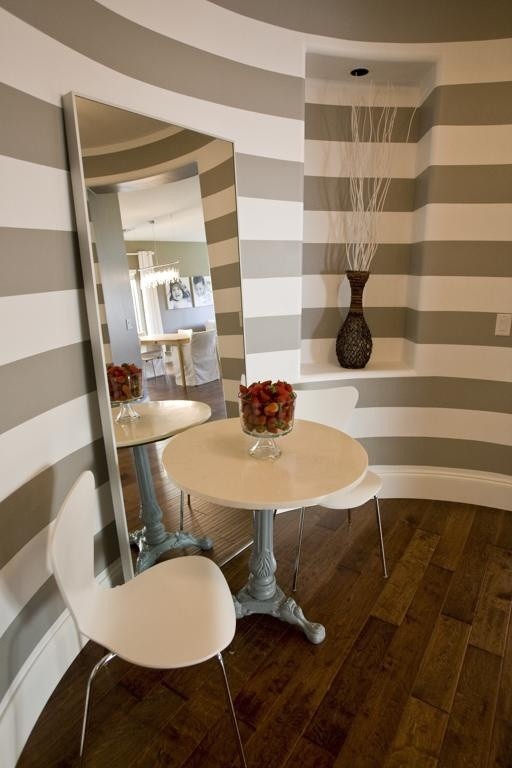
[108,371,144,424]
[238,388,298,461]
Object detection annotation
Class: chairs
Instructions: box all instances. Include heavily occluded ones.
[40,472,248,766]
[287,384,389,593]
[174,330,218,387]
[139,337,157,385]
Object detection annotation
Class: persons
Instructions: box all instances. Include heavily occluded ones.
[169,276,213,309]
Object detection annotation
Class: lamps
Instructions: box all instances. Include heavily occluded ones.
[137,221,182,291]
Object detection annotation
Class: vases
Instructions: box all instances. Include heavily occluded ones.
[336,269,374,369]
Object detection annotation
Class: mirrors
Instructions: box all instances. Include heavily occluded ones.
[57,90,255,584]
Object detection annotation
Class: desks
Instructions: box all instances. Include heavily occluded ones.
[165,415,369,646]
[139,333,190,394]
[111,400,212,573]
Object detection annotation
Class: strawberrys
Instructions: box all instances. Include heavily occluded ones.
[106,363,142,401]
[238,380,297,433]
